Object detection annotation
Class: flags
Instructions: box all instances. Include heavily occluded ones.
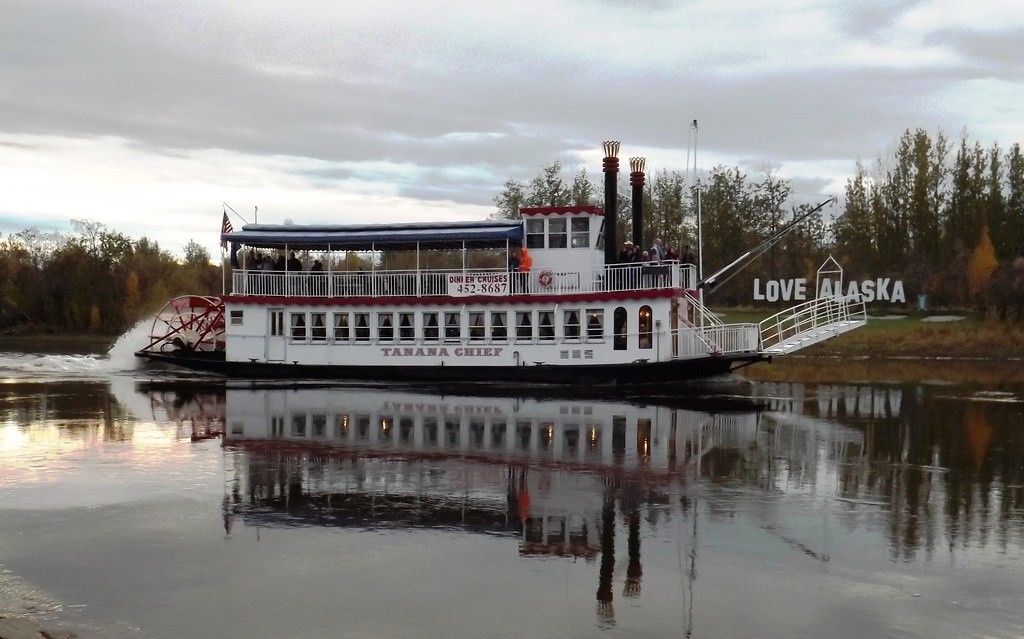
[230,242,242,269]
[219,209,234,252]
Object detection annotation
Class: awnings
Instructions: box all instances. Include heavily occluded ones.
[220,227,524,298]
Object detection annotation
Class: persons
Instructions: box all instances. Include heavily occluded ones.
[245,249,366,297]
[518,248,532,296]
[506,250,520,296]
[617,237,697,290]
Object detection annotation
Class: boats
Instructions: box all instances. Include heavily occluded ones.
[132,119,867,388]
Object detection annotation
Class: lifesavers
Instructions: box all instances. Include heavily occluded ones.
[539,271,552,285]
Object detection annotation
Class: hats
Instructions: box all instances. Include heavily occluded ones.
[624,240,633,245]
